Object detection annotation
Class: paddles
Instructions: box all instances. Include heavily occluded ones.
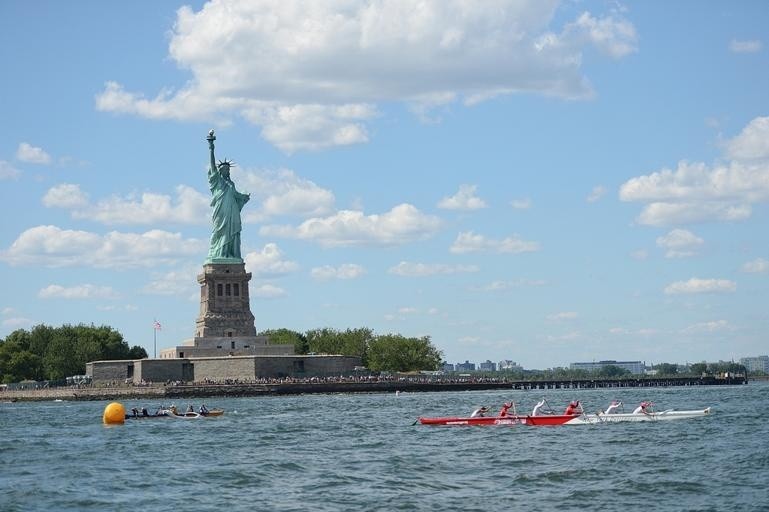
[576,401,592,425]
[650,400,658,422]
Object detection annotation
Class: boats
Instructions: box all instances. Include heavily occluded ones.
[416,407,711,425]
[125,408,224,419]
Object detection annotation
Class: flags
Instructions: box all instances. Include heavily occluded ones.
[154,319,161,330]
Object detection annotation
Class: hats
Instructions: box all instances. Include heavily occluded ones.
[477,401,647,407]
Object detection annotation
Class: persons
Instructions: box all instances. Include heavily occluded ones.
[631,400,655,415]
[605,400,624,414]
[131,407,139,417]
[169,404,177,414]
[499,401,517,417]
[205,142,252,260]
[140,407,147,415]
[469,405,493,418]
[564,401,586,415]
[187,404,193,412]
[199,404,209,413]
[531,398,553,416]
